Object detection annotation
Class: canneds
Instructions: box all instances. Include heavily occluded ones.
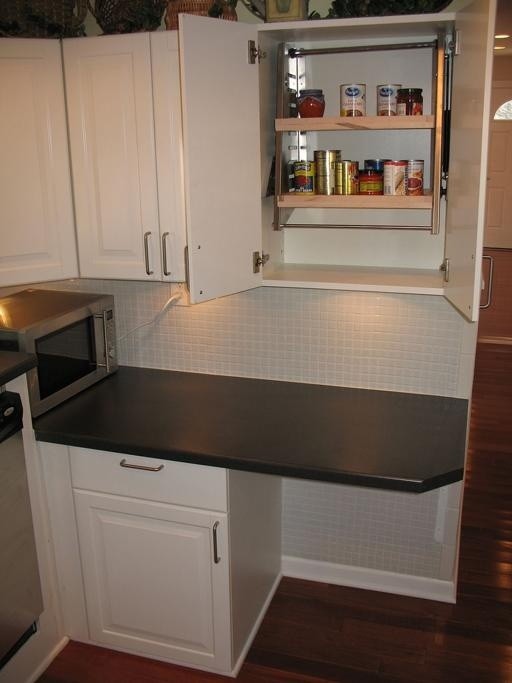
[290,89,298,118]
[294,149,424,195]
[376,84,401,116]
[339,83,367,118]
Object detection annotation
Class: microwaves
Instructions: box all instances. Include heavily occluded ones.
[0,287,120,418]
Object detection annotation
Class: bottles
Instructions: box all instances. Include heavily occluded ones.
[358,170,382,195]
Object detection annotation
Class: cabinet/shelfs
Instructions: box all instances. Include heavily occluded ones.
[0,36,74,293]
[59,30,191,281]
[60,444,281,681]
[177,1,499,324]
